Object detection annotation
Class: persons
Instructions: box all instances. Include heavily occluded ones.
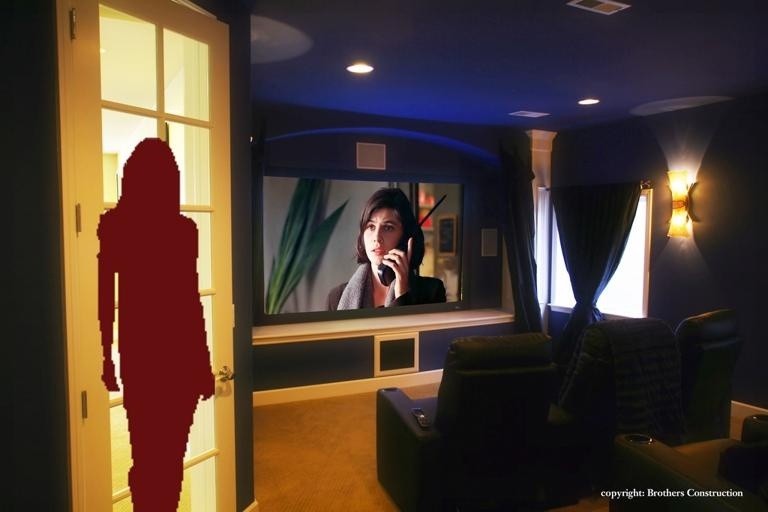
[322,185,447,312]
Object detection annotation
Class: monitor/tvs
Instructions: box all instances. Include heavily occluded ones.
[248,165,463,326]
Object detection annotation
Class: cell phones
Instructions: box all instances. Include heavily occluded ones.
[378,190,450,287]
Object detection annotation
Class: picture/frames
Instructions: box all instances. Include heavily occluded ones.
[439,215,457,256]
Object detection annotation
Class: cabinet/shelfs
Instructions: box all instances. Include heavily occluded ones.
[252,308,517,408]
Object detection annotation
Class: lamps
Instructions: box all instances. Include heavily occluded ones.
[665,170,699,240]
